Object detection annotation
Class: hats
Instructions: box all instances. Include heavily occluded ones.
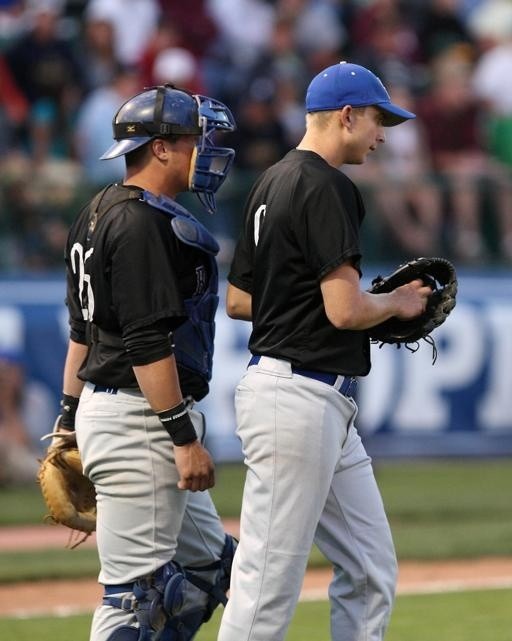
[306,60,417,127]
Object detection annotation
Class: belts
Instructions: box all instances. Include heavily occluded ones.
[94,385,193,410]
[247,355,359,399]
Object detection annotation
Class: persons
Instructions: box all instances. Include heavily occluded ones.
[211,61,433,641]
[1,353,42,487]
[0,1,511,282]
[48,82,241,641]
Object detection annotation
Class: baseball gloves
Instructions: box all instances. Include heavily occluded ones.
[37,432,96,532]
[364,257,457,344]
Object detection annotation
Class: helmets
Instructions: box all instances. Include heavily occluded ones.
[97,84,201,161]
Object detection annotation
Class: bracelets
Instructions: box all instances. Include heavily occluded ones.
[58,392,78,431]
[157,402,198,445]
